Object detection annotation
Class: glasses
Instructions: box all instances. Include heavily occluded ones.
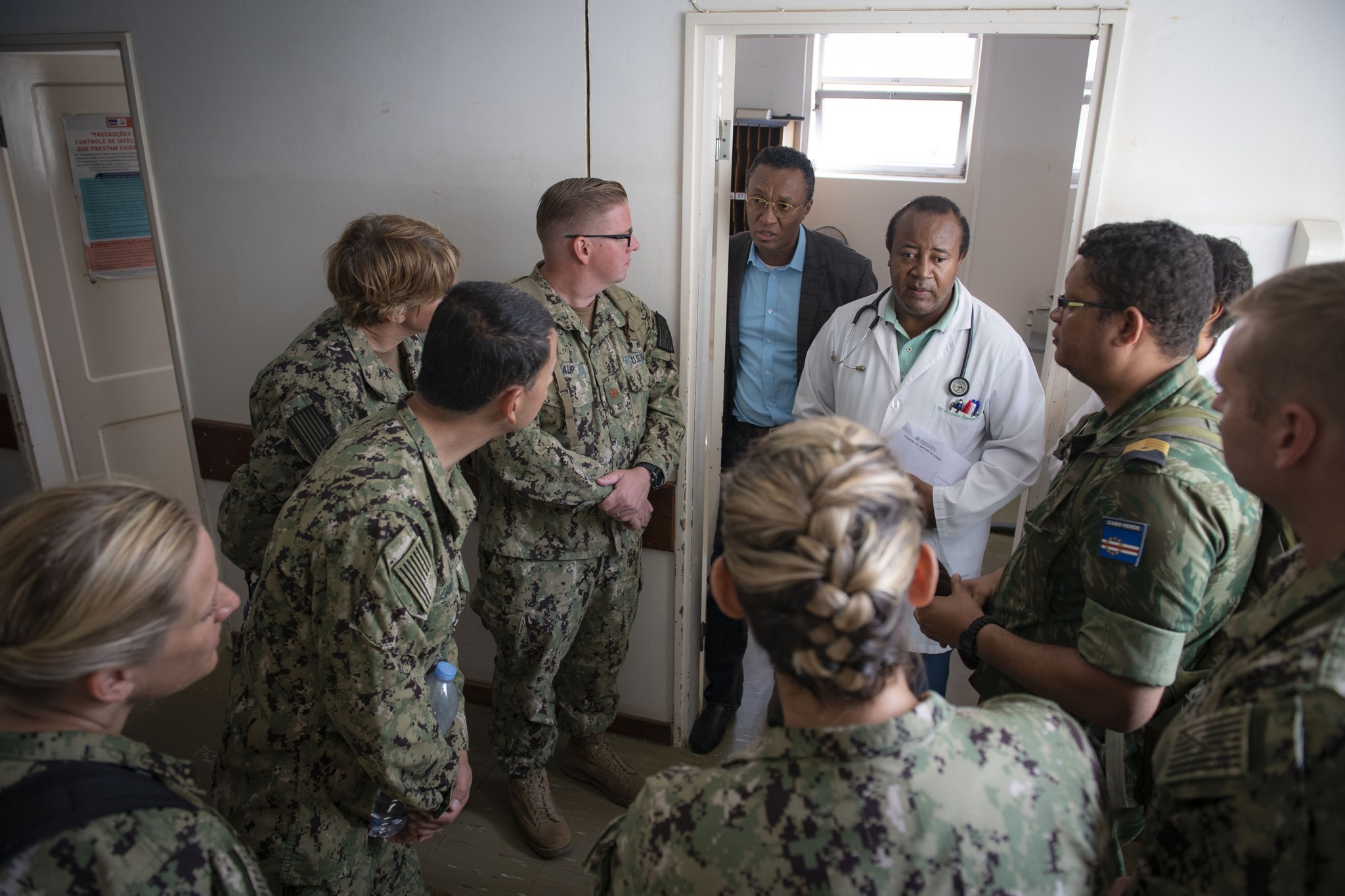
[564,228,633,247]
[1055,293,1156,327]
[744,189,809,220]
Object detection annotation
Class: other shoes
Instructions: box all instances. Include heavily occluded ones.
[563,737,647,807]
[509,769,576,858]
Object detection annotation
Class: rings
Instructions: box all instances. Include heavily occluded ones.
[616,512,622,518]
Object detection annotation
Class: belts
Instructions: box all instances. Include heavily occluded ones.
[732,416,787,439]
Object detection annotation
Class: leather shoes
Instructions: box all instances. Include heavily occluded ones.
[766,683,787,727]
[690,701,737,754]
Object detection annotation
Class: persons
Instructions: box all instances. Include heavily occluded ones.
[473,178,685,861]
[214,214,460,603]
[1114,261,1344,895]
[1,485,275,896]
[790,195,1047,705]
[583,414,1116,896]
[686,147,878,755]
[913,220,1298,851]
[209,279,559,896]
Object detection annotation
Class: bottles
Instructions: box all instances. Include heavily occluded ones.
[368,662,460,837]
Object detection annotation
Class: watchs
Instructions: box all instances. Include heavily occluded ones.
[633,462,664,491]
[959,614,1005,657]
